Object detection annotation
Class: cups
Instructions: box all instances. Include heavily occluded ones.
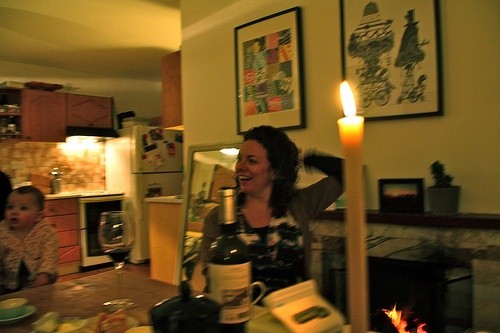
[0,298,29,319]
[51,179,62,194]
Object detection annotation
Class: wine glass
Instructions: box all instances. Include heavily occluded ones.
[98,211,137,310]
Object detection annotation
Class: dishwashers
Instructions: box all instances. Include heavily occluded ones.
[79,196,125,267]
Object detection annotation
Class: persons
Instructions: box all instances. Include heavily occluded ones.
[0,186,58,293]
[199,125,345,306]
[0,170,12,222]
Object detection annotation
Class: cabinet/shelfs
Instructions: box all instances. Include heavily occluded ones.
[40,199,81,264]
[0,88,113,142]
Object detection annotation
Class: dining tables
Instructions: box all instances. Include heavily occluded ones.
[0,269,180,333]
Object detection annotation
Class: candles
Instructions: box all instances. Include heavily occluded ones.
[338,115,369,333]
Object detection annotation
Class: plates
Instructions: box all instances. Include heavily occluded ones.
[0,306,36,325]
[122,117,151,127]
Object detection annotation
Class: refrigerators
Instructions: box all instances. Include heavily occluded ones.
[103,125,183,263]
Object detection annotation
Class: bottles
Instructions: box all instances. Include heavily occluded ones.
[206,186,252,333]
[150,279,220,333]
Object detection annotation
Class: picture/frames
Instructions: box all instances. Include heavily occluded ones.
[234,7,306,135]
[338,0,443,121]
[378,177,425,214]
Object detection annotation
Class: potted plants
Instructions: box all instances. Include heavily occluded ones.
[424,161,461,215]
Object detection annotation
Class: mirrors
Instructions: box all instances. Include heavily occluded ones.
[173,141,243,285]
